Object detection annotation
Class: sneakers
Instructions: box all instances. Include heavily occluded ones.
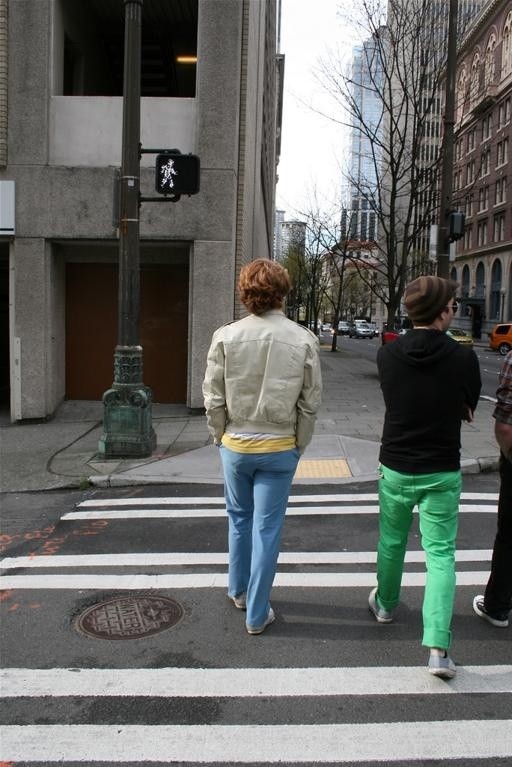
[473,595,509,628]
[233,592,274,635]
[369,587,395,622]
[429,650,457,678]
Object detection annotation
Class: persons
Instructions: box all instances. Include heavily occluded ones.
[474,350,512,628]
[202,257,323,634]
[367,276,481,675]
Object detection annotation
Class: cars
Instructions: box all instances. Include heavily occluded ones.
[307,319,381,339]
[445,327,475,349]
[397,329,412,335]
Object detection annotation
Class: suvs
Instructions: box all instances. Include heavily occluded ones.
[488,322,512,356]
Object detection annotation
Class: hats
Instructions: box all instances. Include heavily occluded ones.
[404,276,460,320]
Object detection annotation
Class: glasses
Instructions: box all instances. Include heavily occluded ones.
[445,302,458,312]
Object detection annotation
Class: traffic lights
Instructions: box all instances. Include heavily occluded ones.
[156,153,200,195]
